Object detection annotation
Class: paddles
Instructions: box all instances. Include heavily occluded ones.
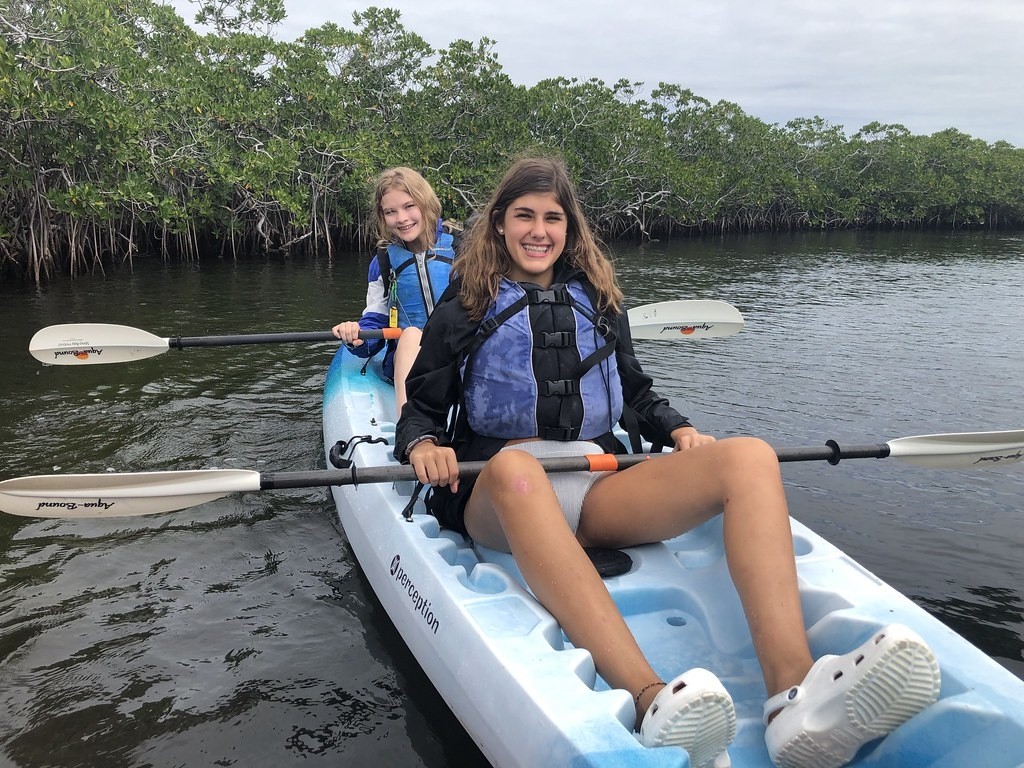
[27,295,748,368]
[1,424,1024,521]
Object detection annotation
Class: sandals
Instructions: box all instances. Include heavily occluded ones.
[763,624,941,768]
[639,668,736,768]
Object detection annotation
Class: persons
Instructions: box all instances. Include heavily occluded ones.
[394,159,943,768]
[332,167,465,420]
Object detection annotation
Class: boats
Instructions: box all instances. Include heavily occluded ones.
[320,334,1024,768]
